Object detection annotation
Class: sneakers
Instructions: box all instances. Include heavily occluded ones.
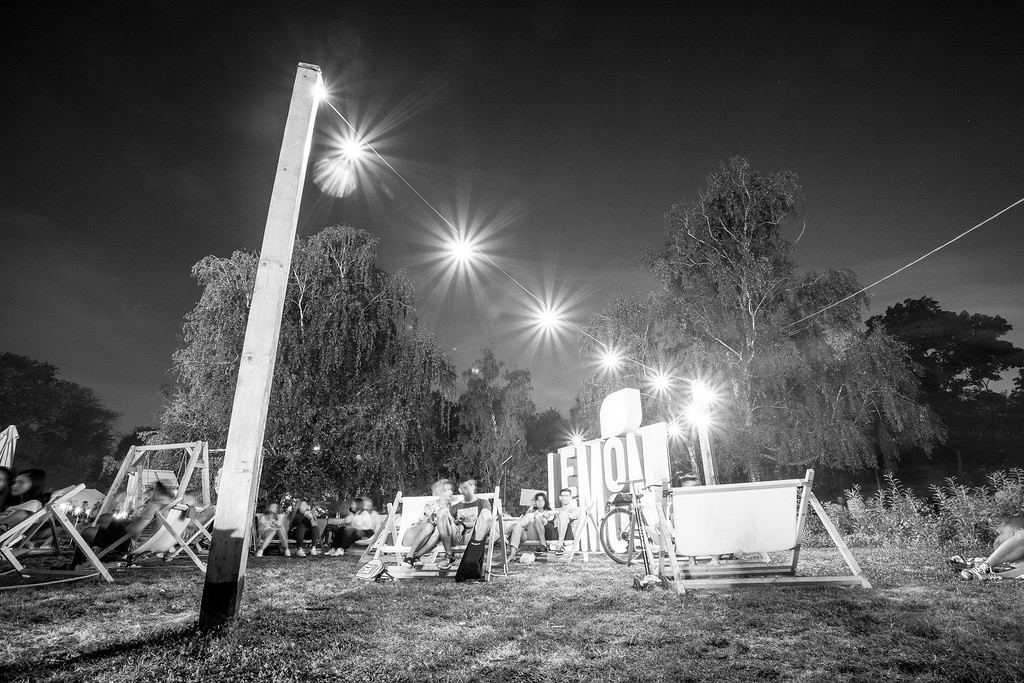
[437,550,455,569]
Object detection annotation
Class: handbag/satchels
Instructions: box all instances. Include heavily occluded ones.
[454,526,490,581]
[605,494,632,512]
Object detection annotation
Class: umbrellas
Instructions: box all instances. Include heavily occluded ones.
[0,423,19,469]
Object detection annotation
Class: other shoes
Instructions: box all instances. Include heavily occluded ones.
[556,546,566,556]
[535,545,549,552]
[297,549,306,557]
[285,548,291,557]
[324,547,335,555]
[257,550,263,557]
[331,548,345,557]
[413,555,423,567]
[403,555,414,567]
[310,548,317,556]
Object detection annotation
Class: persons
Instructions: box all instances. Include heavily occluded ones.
[402,478,492,570]
[496,488,579,562]
[960,528,1024,582]
[0,467,381,570]
[680,472,700,488]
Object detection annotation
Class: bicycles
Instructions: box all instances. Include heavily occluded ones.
[598,469,685,566]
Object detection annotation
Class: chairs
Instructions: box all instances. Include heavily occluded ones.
[0,482,605,592]
